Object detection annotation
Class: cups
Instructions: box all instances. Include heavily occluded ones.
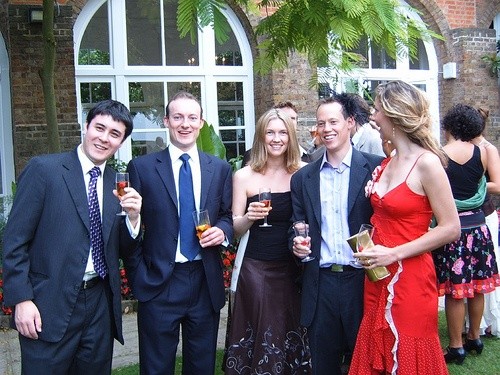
[193,209,211,240]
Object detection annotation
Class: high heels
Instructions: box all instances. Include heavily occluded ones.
[442,346,465,365]
[462,335,485,355]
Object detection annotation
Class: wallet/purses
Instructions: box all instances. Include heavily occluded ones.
[346,231,390,283]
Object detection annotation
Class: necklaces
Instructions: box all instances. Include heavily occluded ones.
[476,137,484,146]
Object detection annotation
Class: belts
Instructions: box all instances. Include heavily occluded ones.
[80,276,102,290]
[325,261,356,274]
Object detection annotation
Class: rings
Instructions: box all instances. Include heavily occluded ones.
[368,260,371,264]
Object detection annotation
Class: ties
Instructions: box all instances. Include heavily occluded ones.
[179,152,200,261]
[87,166,108,279]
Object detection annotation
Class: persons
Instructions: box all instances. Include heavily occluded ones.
[121,90,235,375]
[2,99,143,375]
[230,78,500,375]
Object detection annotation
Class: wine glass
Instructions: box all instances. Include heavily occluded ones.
[258,187,273,227]
[116,173,131,215]
[348,224,375,269]
[293,220,315,262]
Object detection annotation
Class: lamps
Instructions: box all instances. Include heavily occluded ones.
[31,8,44,21]
